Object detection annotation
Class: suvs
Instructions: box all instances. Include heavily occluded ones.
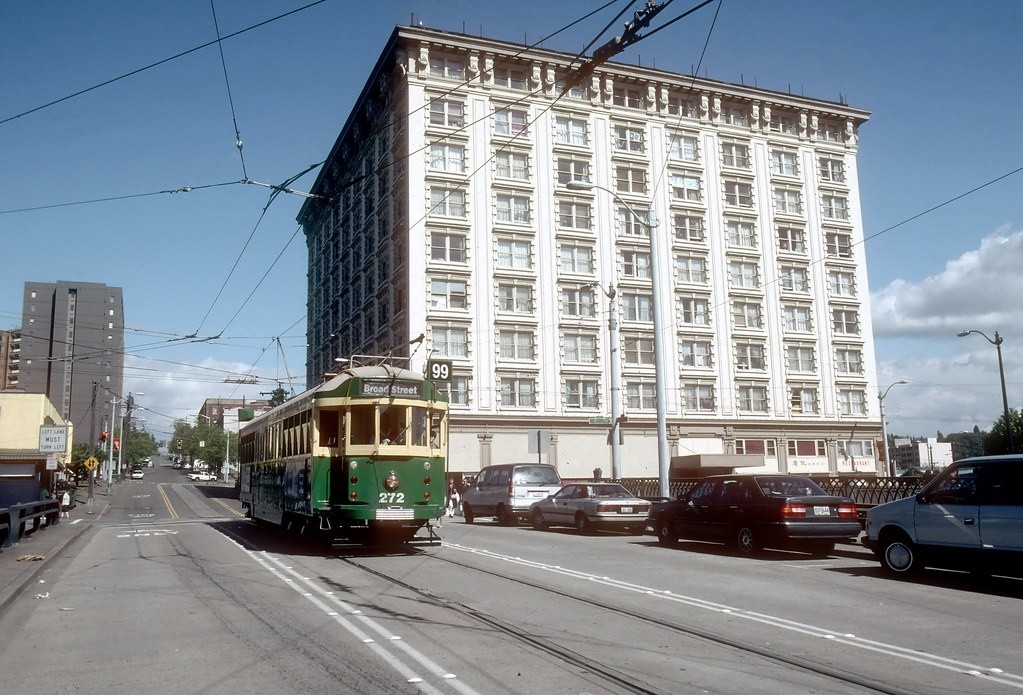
[860,454,1023,581]
[461,463,564,524]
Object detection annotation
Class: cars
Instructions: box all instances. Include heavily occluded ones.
[528,482,652,536]
[648,474,861,554]
[130,456,154,479]
[168,454,217,481]
[899,466,958,486]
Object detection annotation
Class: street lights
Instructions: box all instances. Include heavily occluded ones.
[117,406,144,475]
[582,283,620,478]
[565,180,668,504]
[190,413,211,434]
[877,379,909,487]
[957,328,1012,453]
[107,391,144,488]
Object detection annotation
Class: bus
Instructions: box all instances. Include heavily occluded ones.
[237,363,448,552]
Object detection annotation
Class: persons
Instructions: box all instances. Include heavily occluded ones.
[50,490,58,500]
[370,415,404,445]
[448,477,473,517]
[60,488,71,519]
[430,431,438,450]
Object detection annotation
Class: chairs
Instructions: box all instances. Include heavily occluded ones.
[719,482,741,503]
